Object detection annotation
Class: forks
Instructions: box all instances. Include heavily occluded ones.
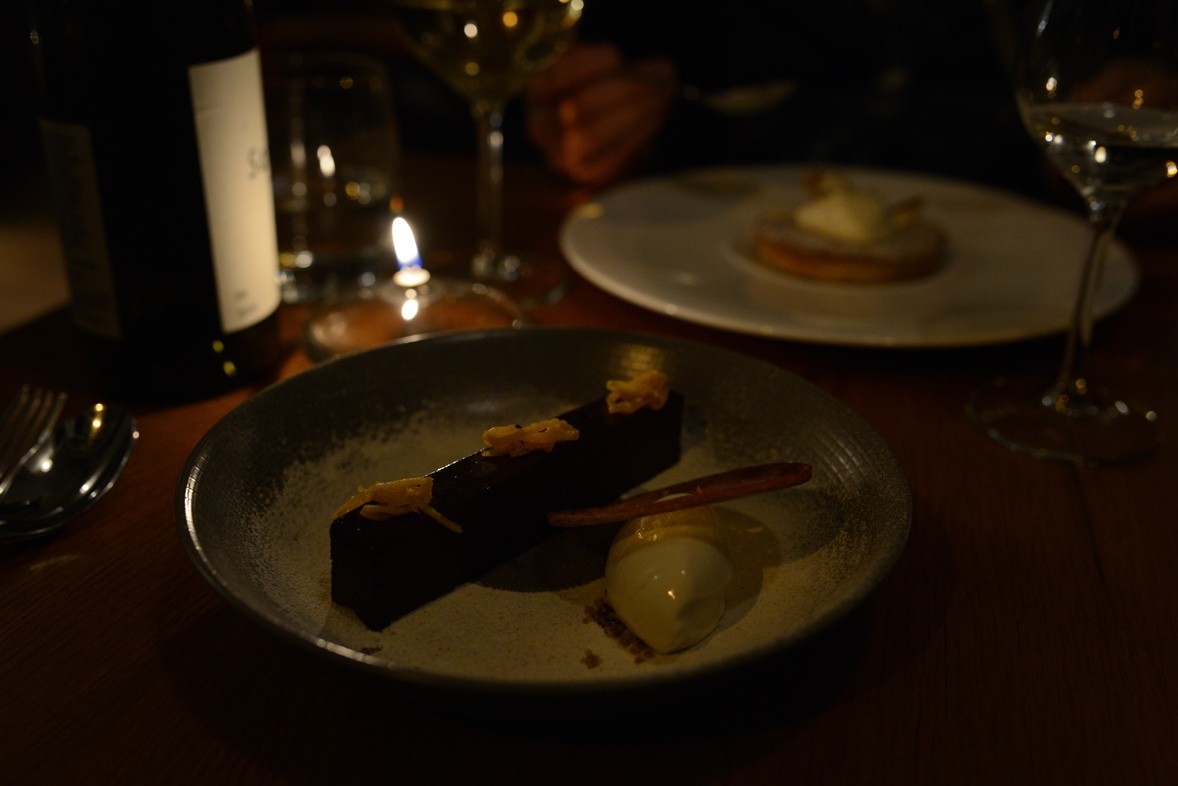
[0,382,74,496]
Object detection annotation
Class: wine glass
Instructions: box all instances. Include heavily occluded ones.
[991,0,1174,475]
[398,0,586,295]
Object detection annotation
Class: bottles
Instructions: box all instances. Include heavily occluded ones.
[16,0,288,400]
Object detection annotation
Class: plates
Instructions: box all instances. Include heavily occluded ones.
[555,165,1136,340]
[181,330,915,691]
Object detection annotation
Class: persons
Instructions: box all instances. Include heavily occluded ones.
[500,2,1095,218]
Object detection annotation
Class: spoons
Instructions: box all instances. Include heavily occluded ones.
[0,403,140,543]
